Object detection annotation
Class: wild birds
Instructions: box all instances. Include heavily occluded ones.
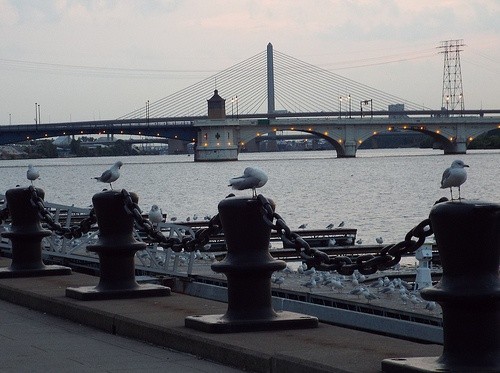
[23,161,41,189]
[89,159,125,190]
[2,204,436,315]
[226,165,269,198]
[439,158,470,202]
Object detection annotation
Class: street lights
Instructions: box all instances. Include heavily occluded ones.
[339,96,342,120]
[35,102,38,130]
[236,96,238,115]
[348,94,351,118]
[38,105,40,124]
[146,100,149,126]
[447,95,449,117]
[231,97,234,115]
[459,95,463,117]
